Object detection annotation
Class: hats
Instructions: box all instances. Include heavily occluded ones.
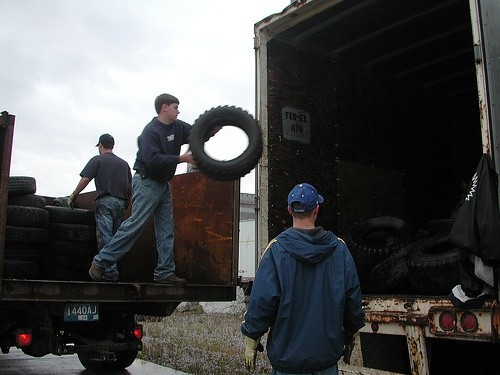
[94,133,115,147]
[288,183,324,212]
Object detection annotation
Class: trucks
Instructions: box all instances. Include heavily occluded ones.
[238,0,500,375]
[0,110,241,374]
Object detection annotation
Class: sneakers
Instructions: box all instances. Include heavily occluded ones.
[89,260,103,281]
[153,273,187,284]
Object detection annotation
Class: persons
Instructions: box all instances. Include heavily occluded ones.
[240,183,366,375]
[68,134,132,281]
[88,93,221,284]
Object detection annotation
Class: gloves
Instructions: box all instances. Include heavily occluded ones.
[241,335,264,373]
[343,340,355,366]
[67,191,80,210]
